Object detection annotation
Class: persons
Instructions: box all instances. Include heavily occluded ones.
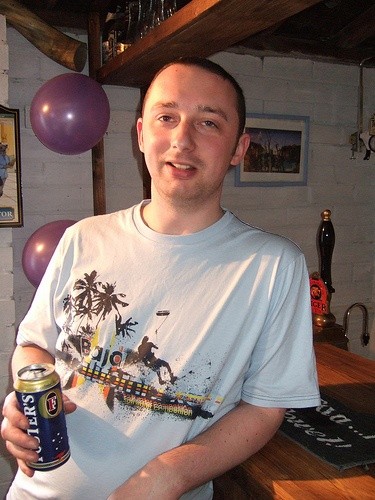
[1,56,322,500]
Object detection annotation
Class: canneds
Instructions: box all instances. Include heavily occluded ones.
[12,363,71,471]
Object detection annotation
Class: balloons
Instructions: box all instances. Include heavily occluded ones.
[30,73,110,155]
[22,220,79,288]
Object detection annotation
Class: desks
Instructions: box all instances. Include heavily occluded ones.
[226,339,375,500]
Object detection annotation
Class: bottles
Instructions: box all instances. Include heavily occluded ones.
[102,0,177,65]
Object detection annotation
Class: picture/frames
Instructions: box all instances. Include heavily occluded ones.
[233,113,311,187]
[0,104,24,229]
